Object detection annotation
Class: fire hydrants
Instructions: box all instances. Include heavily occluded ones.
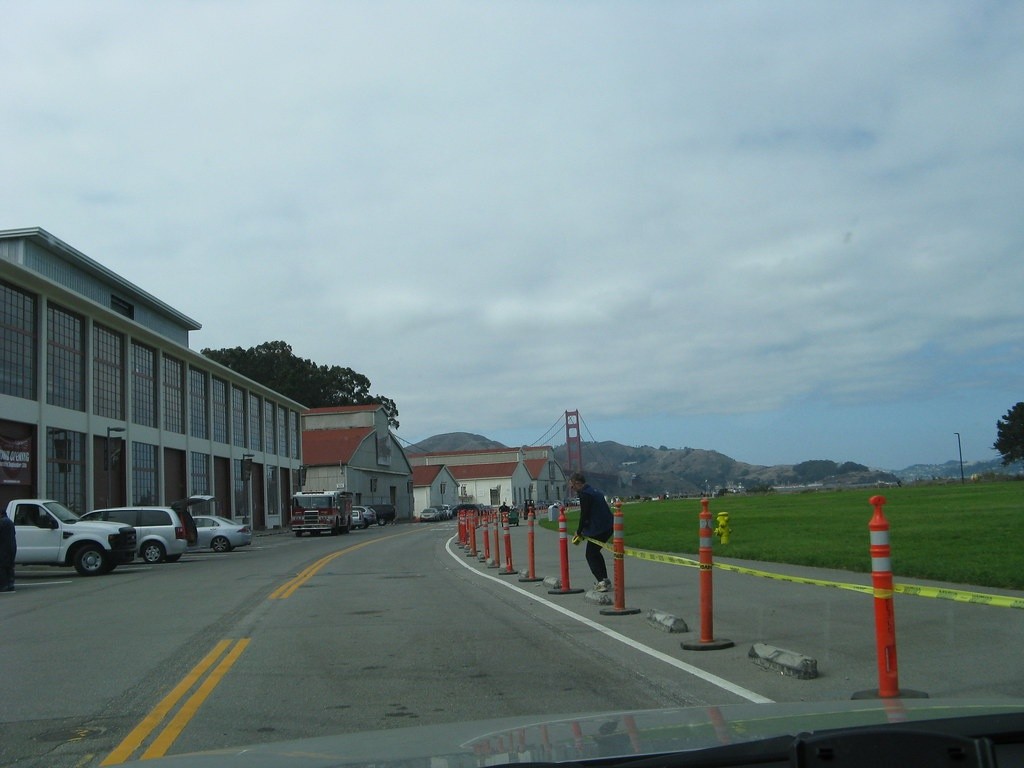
[715,511,734,546]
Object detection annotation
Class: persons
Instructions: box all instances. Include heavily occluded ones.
[570,473,614,592]
[499,502,510,527]
[0,508,17,593]
[510,501,518,526]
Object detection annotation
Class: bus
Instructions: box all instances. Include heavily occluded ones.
[288,490,353,538]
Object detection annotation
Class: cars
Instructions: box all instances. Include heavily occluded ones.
[500,494,621,528]
[435,501,495,521]
[420,507,442,522]
[350,508,365,530]
[191,514,252,553]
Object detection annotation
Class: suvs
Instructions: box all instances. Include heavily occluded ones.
[366,503,397,527]
[352,504,377,529]
[76,495,217,566]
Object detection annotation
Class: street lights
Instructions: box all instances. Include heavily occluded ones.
[954,433,965,484]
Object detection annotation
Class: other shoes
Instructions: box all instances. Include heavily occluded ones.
[593,579,612,592]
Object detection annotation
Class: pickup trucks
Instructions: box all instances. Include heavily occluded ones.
[5,499,138,578]
